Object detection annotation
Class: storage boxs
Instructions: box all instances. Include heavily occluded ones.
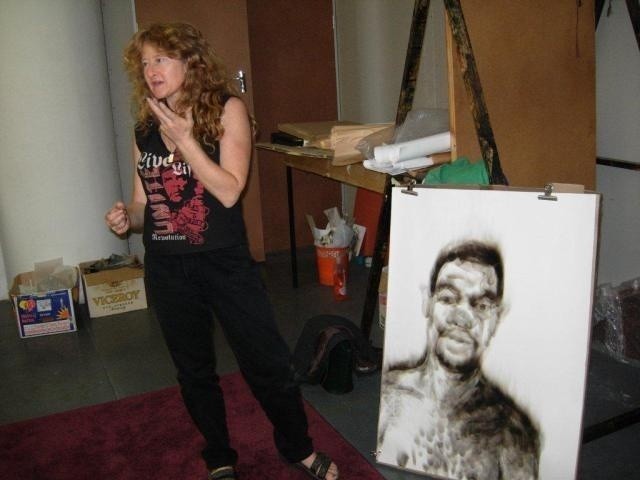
[11,271,78,338]
[76,257,146,321]
[353,455,386,457]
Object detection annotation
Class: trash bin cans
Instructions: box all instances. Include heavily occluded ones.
[315,243,350,288]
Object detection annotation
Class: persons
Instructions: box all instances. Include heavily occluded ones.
[105,22,340,480]
[381,240,542,480]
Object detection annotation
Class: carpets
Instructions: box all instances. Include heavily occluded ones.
[0,371,384,480]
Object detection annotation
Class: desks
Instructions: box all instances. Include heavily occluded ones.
[282,147,393,289]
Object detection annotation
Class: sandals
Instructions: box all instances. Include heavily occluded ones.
[282,448,338,480]
[210,467,236,476]
